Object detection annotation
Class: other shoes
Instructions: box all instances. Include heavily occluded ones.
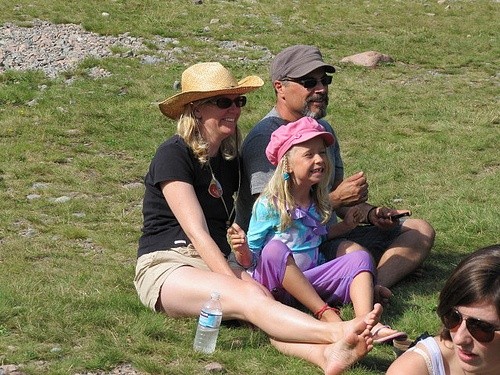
[393,331,434,356]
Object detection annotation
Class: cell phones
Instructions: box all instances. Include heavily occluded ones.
[383,211,410,220]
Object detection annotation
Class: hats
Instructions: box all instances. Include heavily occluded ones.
[266,116,335,167]
[159,62,265,119]
[272,45,336,88]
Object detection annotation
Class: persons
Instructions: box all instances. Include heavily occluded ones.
[134,43,383,375]
[238,45,436,298]
[387,244,500,375]
[226,118,408,346]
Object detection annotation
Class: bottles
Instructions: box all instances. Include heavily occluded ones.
[192,290,223,354]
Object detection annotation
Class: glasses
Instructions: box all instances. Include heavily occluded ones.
[440,306,500,343]
[278,75,333,88]
[199,96,246,109]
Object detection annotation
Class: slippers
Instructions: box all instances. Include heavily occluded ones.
[369,322,408,344]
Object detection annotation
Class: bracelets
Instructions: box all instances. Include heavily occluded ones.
[367,206,378,224]
